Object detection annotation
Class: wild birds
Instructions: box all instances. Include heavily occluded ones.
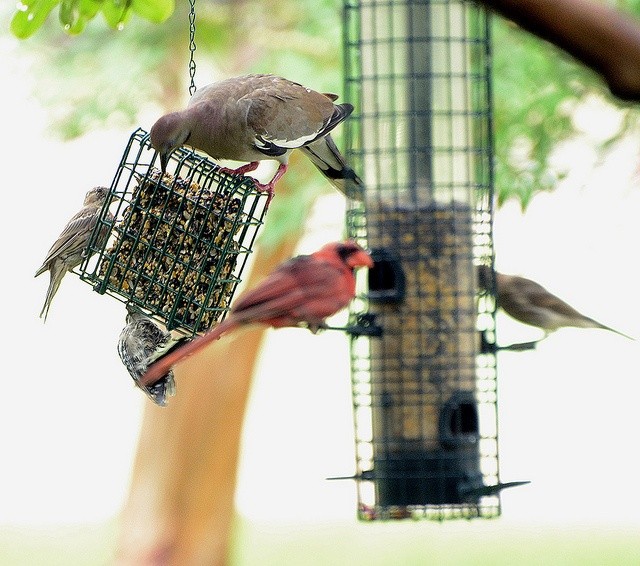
[139,242,374,387]
[117,305,175,407]
[35,187,118,323]
[150,73,365,216]
[476,265,636,349]
